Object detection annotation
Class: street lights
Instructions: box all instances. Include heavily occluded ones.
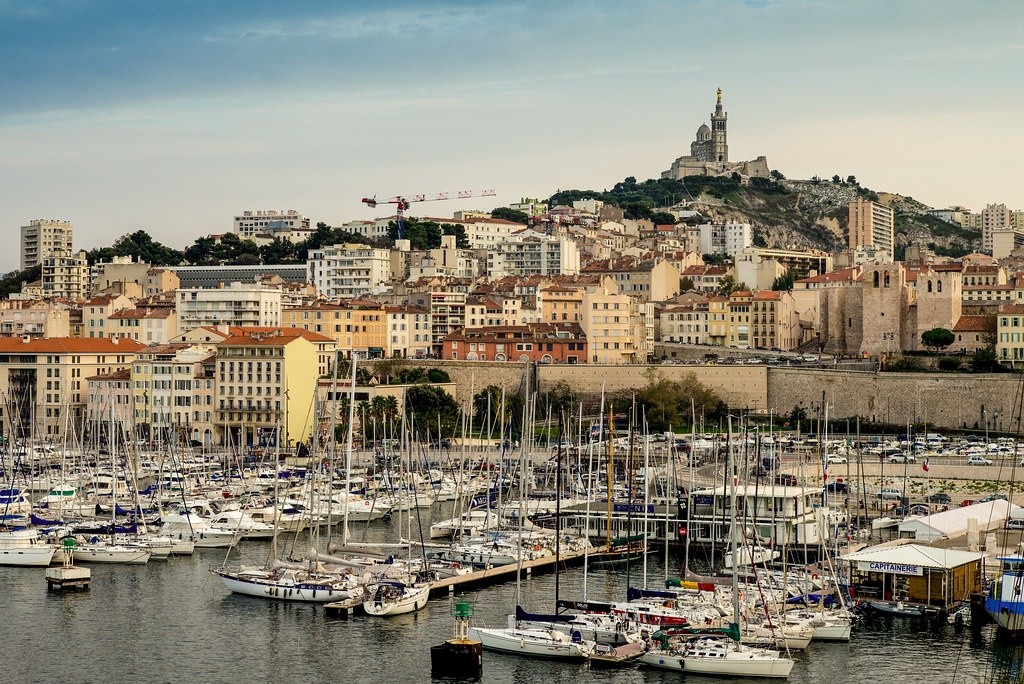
[984,410,988,452]
[751,400,760,457]
[629,406,632,438]
[817,400,826,458]
[913,403,917,461]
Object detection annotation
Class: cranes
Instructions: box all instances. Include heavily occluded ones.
[363,188,498,240]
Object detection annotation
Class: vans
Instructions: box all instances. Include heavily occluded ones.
[880,448,903,457]
[763,457,780,470]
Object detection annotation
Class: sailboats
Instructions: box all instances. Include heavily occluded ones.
[1,353,536,568]
[207,351,651,617]
[467,409,1024,684]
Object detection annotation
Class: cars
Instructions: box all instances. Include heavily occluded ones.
[959,499,979,507]
[754,464,767,476]
[967,456,993,466]
[552,442,574,451]
[895,505,930,515]
[775,474,797,486]
[662,354,820,365]
[824,482,851,494]
[598,431,797,451]
[980,494,1007,503]
[924,493,952,505]
[429,442,450,450]
[823,454,848,465]
[128,439,149,446]
[876,488,902,501]
[888,453,916,464]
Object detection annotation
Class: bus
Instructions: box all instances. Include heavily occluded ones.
[381,439,400,449]
